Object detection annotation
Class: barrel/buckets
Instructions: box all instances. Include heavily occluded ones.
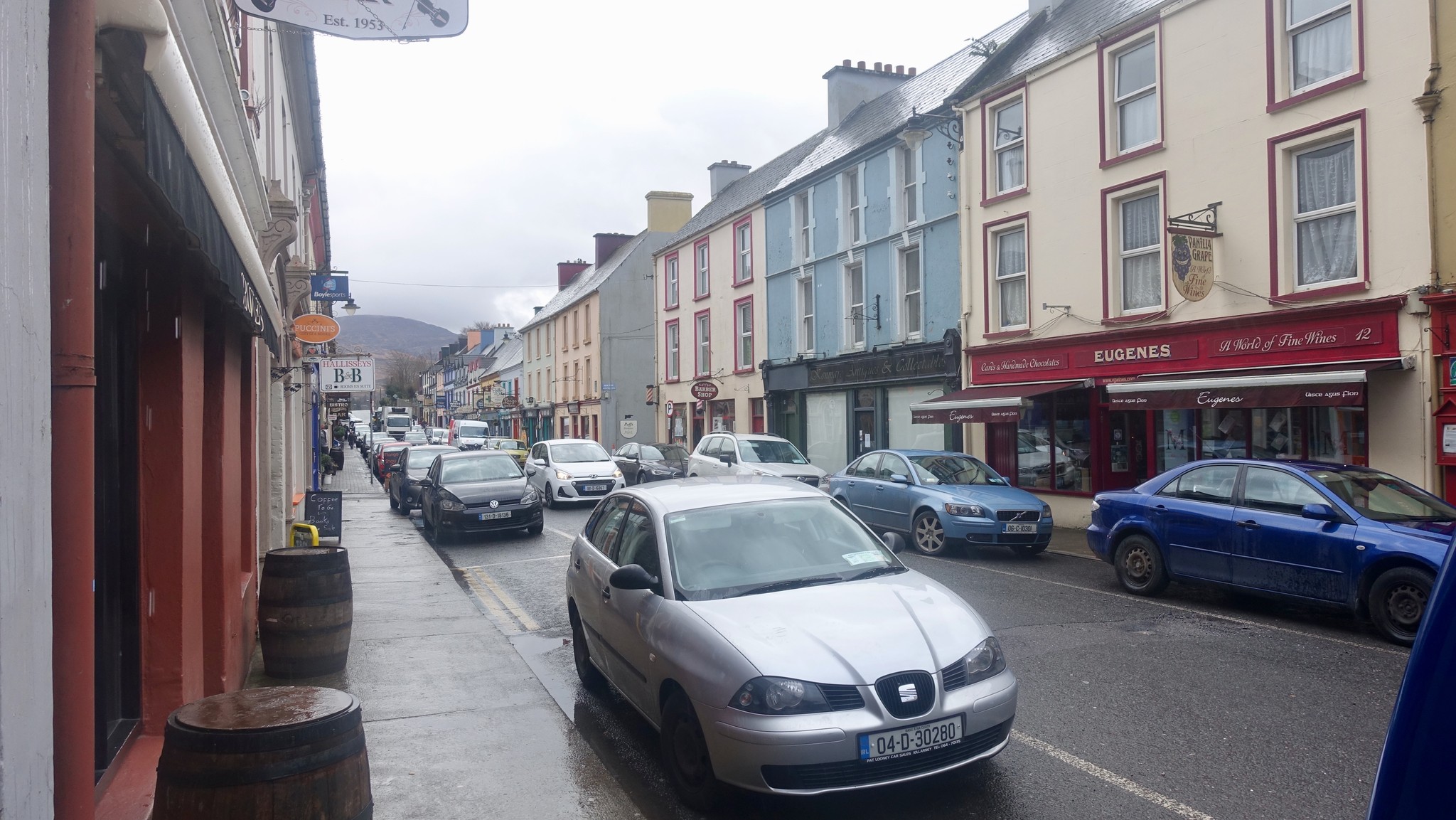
[149,684,375,820]
[258,544,353,679]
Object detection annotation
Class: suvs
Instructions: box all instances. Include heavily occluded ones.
[687,429,831,500]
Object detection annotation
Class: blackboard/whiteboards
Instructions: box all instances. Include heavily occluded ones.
[305,490,343,537]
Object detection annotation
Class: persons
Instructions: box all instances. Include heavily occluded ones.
[343,424,349,440]
[348,431,356,450]
[320,424,335,474]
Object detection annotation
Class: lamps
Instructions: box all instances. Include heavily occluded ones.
[895,116,932,153]
[341,299,361,317]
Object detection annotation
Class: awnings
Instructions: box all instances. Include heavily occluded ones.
[910,378,1095,424]
[1106,355,1415,411]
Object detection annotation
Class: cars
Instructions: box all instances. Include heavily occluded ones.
[418,450,545,537]
[609,441,693,488]
[524,439,628,510]
[564,473,1019,803]
[1086,454,1456,645]
[341,406,530,516]
[828,448,1056,556]
